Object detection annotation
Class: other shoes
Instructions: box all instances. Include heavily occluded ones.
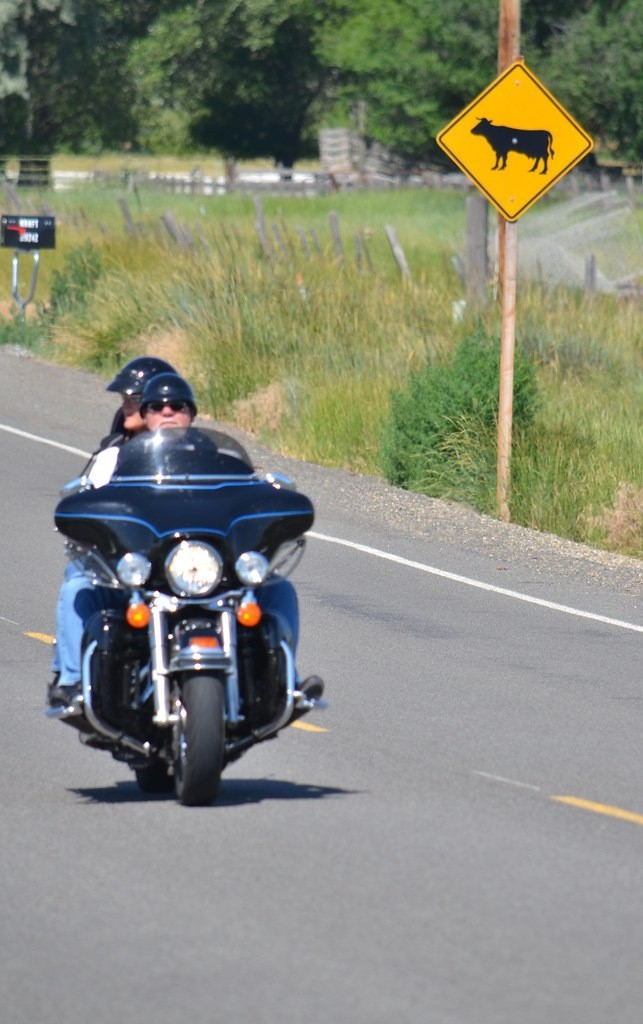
[281,676,324,727]
[50,680,82,707]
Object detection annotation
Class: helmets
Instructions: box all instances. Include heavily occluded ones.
[141,373,194,403]
[106,358,177,395]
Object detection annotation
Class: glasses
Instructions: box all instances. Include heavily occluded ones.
[123,393,142,402]
[148,401,187,411]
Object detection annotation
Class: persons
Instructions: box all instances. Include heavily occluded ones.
[44,356,325,728]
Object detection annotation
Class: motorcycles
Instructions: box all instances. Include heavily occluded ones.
[51,423,317,810]
